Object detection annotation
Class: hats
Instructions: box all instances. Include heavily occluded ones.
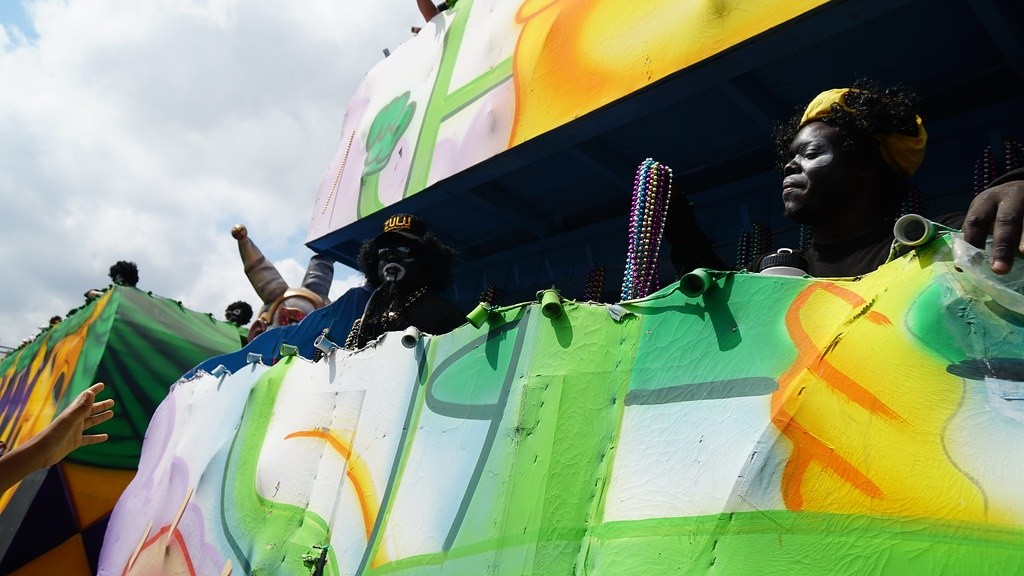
[374,213,428,248]
[799,89,928,162]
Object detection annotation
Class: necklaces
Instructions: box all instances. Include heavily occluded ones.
[479,285,501,306]
[583,263,608,302]
[380,286,428,324]
[620,158,673,303]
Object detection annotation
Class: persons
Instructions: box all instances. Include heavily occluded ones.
[0,382,114,493]
[226,302,253,327]
[84,261,139,297]
[346,214,467,349]
[771,75,1024,275]
